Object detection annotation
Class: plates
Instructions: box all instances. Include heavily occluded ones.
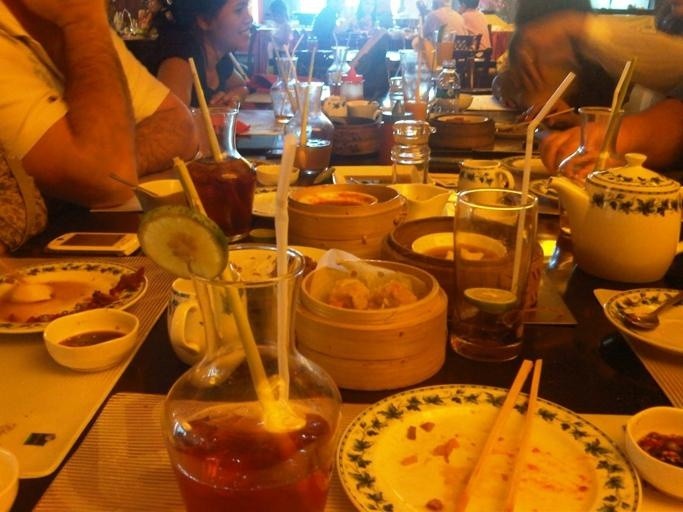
[603,286,682,359]
[1,265,150,338]
[251,184,278,219]
[337,388,643,511]
[332,165,422,185]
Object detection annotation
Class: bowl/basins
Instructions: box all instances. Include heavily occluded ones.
[461,88,518,124]
[256,164,300,188]
[287,184,407,260]
[41,306,138,375]
[431,113,495,150]
[618,405,682,501]
[390,182,452,218]
[328,119,384,156]
[293,258,448,389]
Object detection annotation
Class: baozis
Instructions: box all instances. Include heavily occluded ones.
[329,278,417,311]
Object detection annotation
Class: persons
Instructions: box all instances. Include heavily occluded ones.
[137,0,254,106]
[107,0,175,42]
[509,0,682,176]
[492,0,612,109]
[259,0,491,99]
[0,0,199,212]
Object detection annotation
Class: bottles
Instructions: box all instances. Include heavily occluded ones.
[159,243,342,511]
[186,106,258,242]
[547,107,627,280]
[268,32,462,137]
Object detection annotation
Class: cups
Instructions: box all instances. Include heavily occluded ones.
[449,188,539,362]
[166,275,214,366]
[460,158,515,189]
[133,180,191,210]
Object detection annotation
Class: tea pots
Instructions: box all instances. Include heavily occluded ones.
[545,152,682,285]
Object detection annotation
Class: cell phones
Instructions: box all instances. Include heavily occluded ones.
[44,231,138,257]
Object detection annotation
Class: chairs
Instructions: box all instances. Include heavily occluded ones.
[447,33,495,50]
[452,48,493,89]
